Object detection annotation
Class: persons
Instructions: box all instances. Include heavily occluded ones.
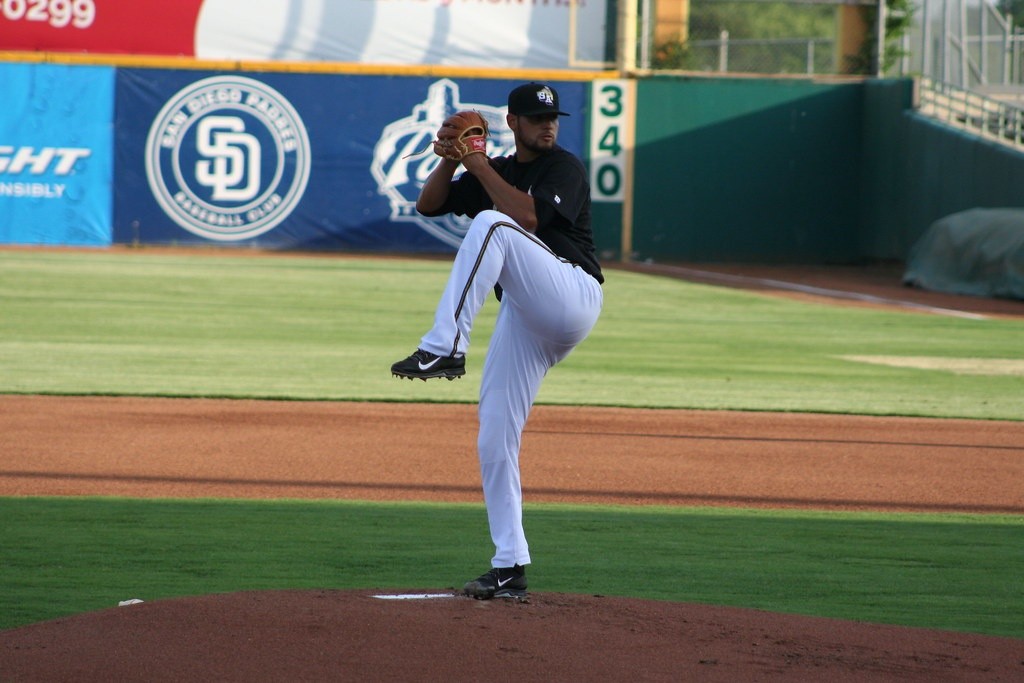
[391,81,605,599]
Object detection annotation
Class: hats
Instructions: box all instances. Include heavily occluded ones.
[508,81,570,116]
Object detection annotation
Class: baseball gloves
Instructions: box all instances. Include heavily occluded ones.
[434,110,488,160]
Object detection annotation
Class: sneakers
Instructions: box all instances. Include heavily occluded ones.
[463,565,528,599]
[391,349,467,382]
